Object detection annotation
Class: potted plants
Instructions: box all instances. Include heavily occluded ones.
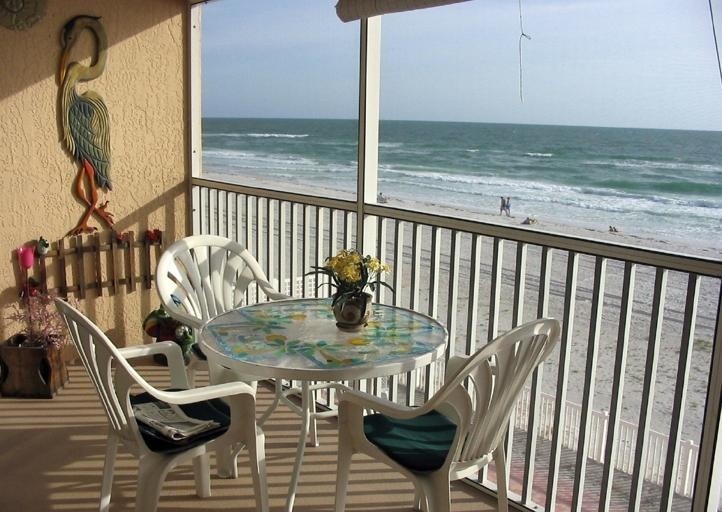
[141,295,196,366]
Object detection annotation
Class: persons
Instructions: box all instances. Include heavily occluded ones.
[498,196,506,217]
[377,192,387,204]
[505,197,512,217]
[521,216,535,225]
[612,227,618,232]
[608,225,613,231]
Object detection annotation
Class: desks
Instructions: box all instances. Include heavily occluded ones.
[198,297,448,512]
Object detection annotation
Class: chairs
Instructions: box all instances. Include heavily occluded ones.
[156,234,320,449]
[334,317,560,512]
[53,297,270,511]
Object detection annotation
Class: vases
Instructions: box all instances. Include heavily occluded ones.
[0,332,69,399]
[330,292,372,331]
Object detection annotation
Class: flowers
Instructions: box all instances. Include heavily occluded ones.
[3,244,83,348]
[304,248,398,306]
